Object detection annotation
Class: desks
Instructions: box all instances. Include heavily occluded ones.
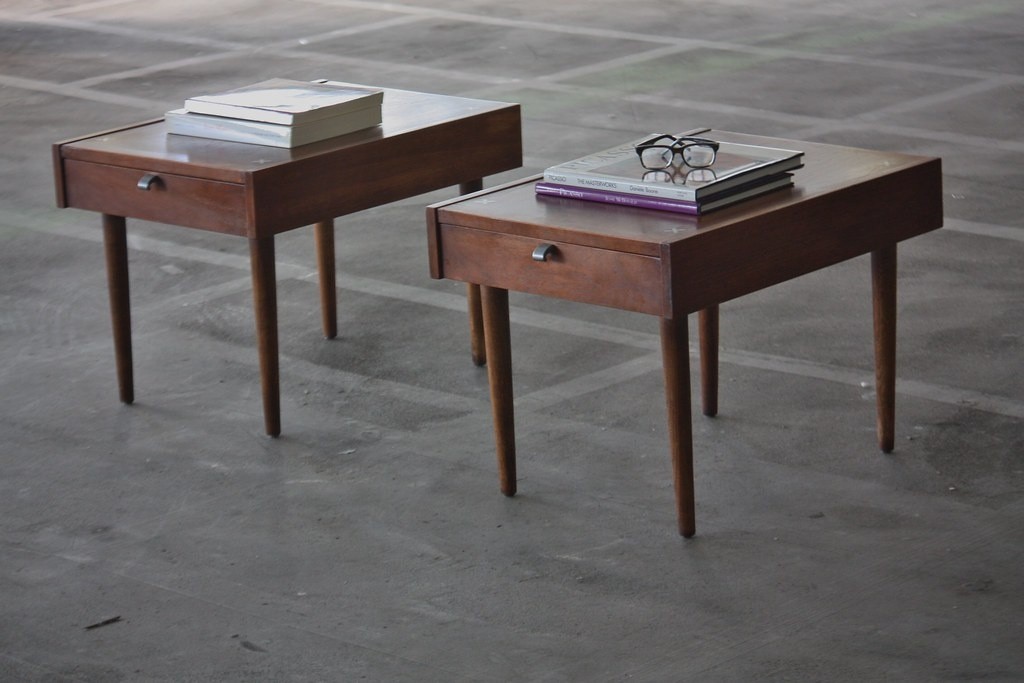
[51,78,523,437]
[425,129,943,540]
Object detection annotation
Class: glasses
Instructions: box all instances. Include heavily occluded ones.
[642,157,717,188]
[635,134,719,170]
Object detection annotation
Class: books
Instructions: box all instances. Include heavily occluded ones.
[534,133,804,214]
[162,76,384,149]
